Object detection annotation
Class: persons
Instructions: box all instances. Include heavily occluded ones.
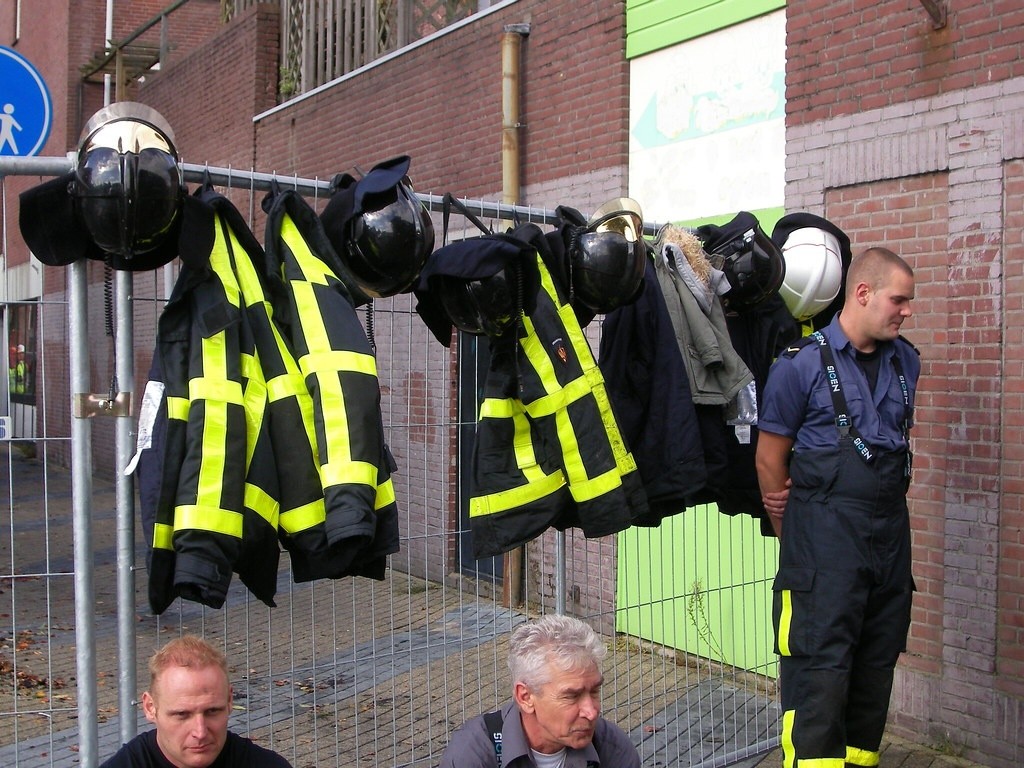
[97,636,292,768]
[438,614,641,768]
[755,248,920,768]
[9,344,29,394]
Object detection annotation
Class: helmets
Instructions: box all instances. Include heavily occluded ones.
[572,197,646,315]
[779,225,844,323]
[712,224,784,318]
[332,176,436,298]
[69,102,188,272]
[425,234,535,337]
